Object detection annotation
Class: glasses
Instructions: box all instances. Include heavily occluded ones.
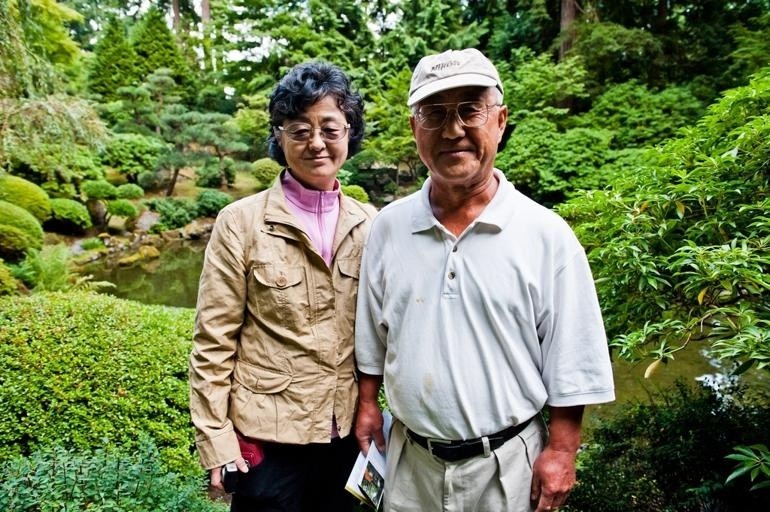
[411,100,497,131]
[279,121,351,144]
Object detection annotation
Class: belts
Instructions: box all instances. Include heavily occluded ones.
[407,415,535,462]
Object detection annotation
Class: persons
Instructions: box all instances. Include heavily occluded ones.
[186,57,379,512]
[352,47,619,512]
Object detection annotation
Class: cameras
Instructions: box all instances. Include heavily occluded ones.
[221,460,251,493]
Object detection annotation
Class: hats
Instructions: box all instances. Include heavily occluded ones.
[407,47,504,106]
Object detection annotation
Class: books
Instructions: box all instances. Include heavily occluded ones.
[341,406,397,512]
